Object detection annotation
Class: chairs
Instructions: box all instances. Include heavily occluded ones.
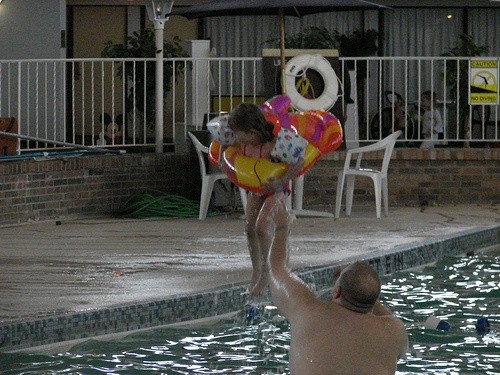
[335,130,401,218]
[188,131,252,220]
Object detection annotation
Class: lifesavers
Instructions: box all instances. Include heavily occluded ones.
[206,95,347,190]
[281,54,341,111]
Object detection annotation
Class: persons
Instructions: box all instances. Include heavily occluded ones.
[414,90,442,148]
[266,201,409,374]
[228,102,295,297]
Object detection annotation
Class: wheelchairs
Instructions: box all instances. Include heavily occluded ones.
[370,91,425,148]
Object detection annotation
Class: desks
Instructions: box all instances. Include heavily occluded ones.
[286,174,334,218]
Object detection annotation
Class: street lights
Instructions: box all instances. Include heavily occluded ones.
[143,0,175,154]
